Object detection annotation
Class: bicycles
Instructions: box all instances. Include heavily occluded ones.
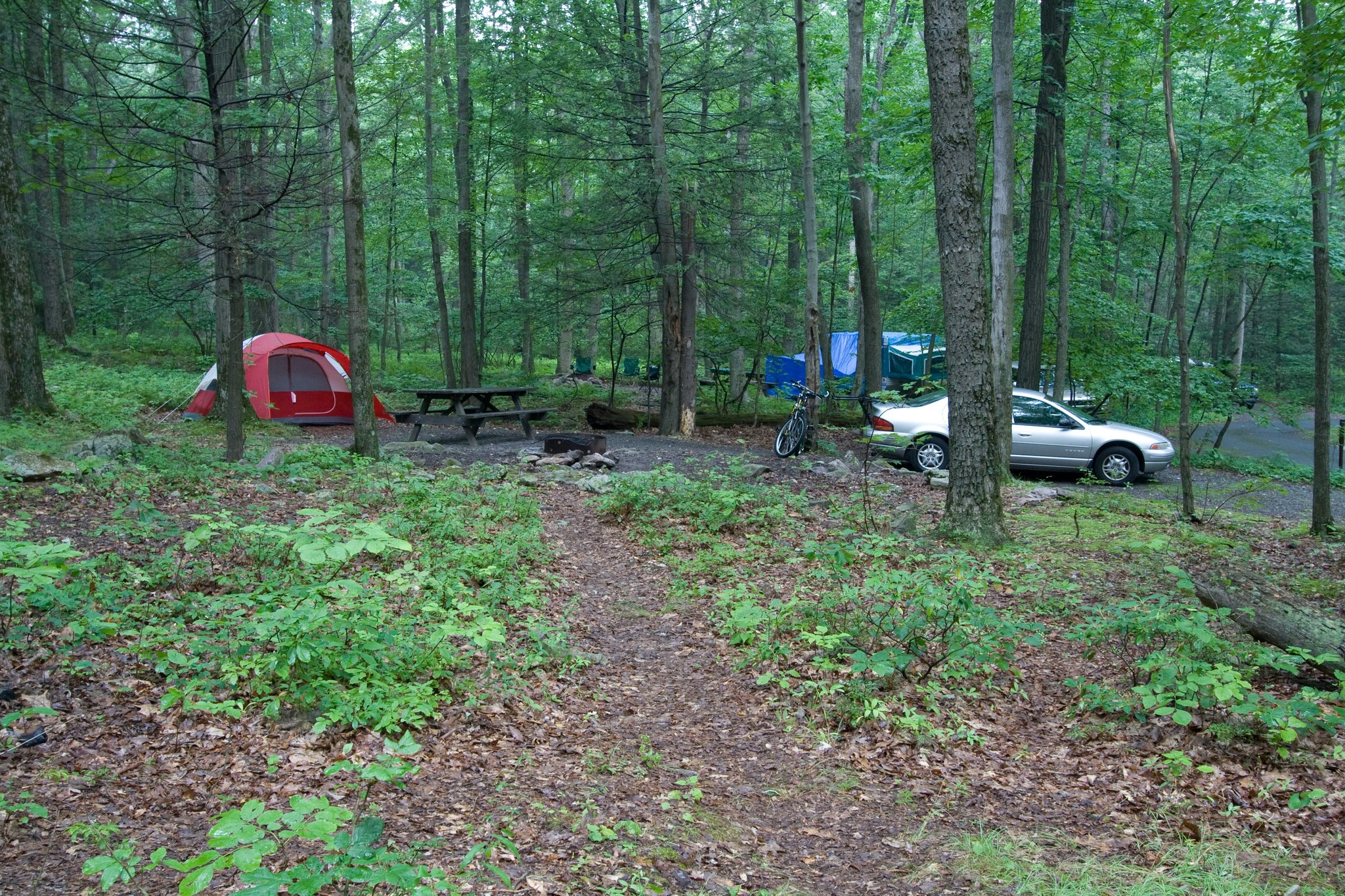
[773,378,829,458]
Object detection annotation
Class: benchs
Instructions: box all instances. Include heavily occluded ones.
[384,407,558,446]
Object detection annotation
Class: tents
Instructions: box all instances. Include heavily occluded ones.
[766,331,946,401]
[181,331,396,425]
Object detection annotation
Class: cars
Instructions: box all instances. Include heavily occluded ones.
[1167,355,1261,409]
[861,384,1175,488]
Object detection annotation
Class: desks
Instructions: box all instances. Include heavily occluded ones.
[401,387,538,447]
